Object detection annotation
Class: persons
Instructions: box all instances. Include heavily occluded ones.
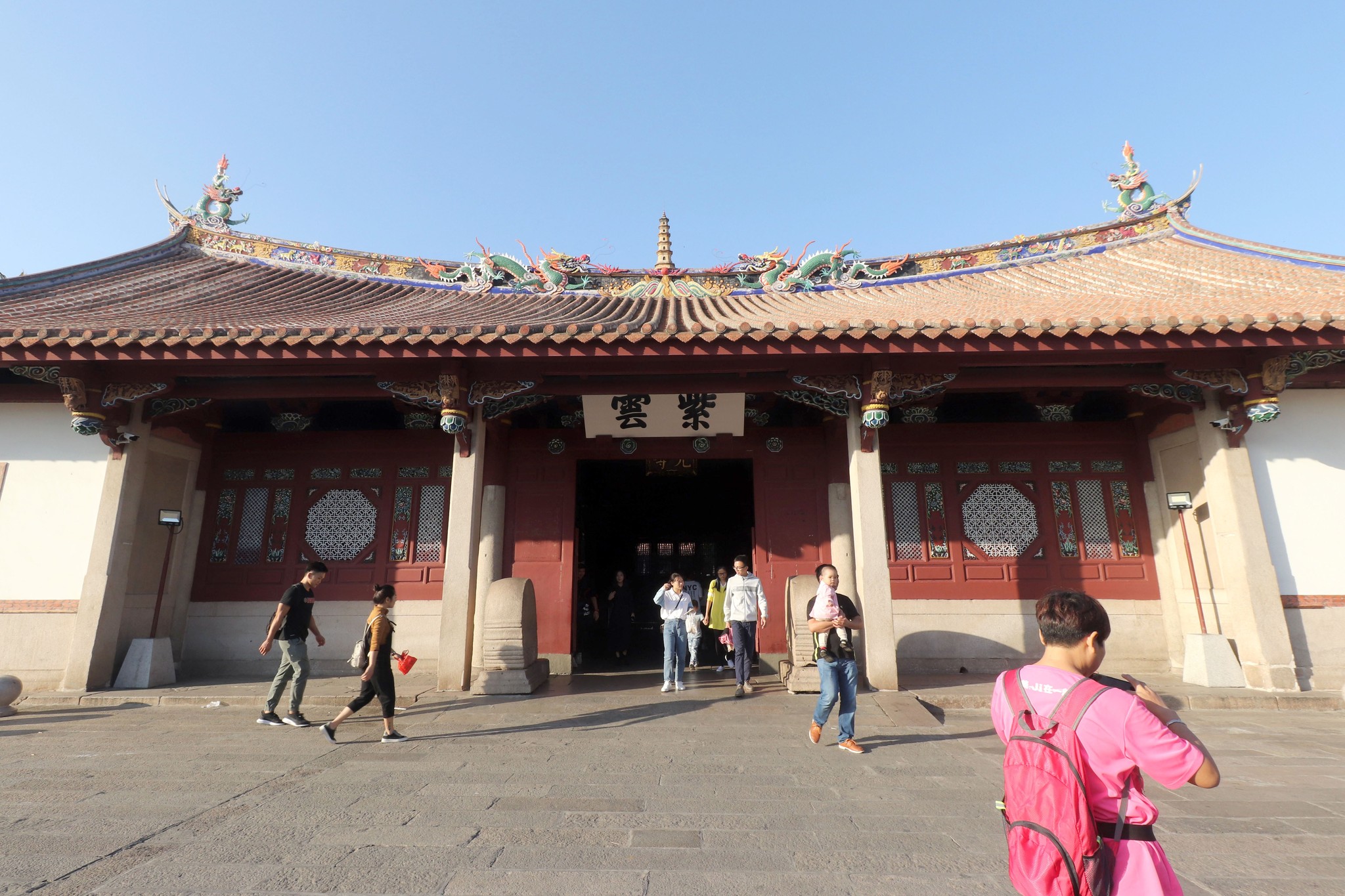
[682,571,703,614]
[807,564,863,753]
[652,573,696,692]
[723,555,768,697]
[990,589,1220,896]
[809,565,853,662]
[319,583,409,744]
[604,569,635,659]
[685,599,707,670]
[258,561,328,727]
[571,567,599,667]
[705,567,734,671]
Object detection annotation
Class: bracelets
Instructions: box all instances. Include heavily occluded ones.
[1163,719,1185,727]
[594,609,598,611]
[390,651,396,656]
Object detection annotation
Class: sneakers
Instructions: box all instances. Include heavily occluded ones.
[380,729,408,743]
[256,710,285,726]
[324,722,336,743]
[281,710,310,727]
[661,680,672,692]
[675,681,686,690]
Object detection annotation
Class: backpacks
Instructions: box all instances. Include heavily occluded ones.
[266,583,300,636]
[347,614,387,668]
[996,666,1134,896]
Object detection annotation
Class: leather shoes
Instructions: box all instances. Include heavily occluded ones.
[743,680,754,691]
[735,684,745,696]
[838,739,864,754]
[809,718,822,744]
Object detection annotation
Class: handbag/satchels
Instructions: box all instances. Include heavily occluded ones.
[660,623,664,634]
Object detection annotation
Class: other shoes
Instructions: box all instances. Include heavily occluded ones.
[716,665,725,672]
[820,647,835,663]
[839,640,855,652]
[689,663,695,670]
[694,664,699,667]
[572,657,578,667]
[575,652,582,664]
[725,655,734,668]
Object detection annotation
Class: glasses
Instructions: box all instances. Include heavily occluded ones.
[732,566,744,570]
[718,572,726,576]
